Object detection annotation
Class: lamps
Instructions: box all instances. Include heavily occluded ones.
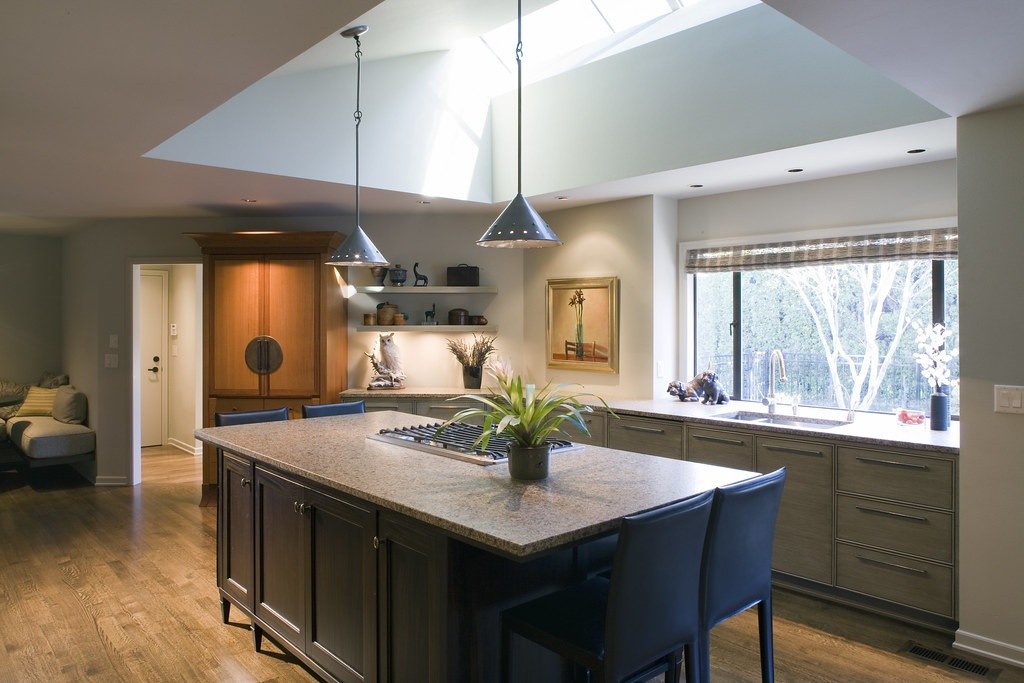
[322,22,390,267]
[474,1,565,251]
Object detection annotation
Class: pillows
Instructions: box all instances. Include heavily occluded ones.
[15,384,63,416]
[38,372,67,387]
[51,384,87,424]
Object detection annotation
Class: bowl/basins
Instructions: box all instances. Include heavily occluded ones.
[448,308,469,325]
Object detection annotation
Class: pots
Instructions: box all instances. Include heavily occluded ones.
[377,301,398,325]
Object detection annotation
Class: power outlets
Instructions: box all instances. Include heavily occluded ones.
[104,354,119,368]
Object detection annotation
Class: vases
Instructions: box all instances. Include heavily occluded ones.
[462,366,483,389]
[930,383,949,431]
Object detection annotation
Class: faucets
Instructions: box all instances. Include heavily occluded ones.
[832,383,857,422]
[757,350,788,412]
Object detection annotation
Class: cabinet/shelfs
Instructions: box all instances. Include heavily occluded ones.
[348,397,960,632]
[214,448,457,682]
[353,286,499,335]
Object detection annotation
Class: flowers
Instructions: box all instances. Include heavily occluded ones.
[907,314,956,391]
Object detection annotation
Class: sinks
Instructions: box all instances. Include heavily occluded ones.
[754,418,842,429]
[714,414,769,422]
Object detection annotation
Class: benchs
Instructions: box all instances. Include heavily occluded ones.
[0,370,94,491]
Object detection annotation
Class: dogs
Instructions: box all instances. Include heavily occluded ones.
[667,373,703,402]
[701,371,730,405]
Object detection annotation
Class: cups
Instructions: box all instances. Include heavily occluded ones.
[389,264,407,287]
[364,313,376,325]
[394,313,407,325]
[370,266,389,286]
[469,316,484,324]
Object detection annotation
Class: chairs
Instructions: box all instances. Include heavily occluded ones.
[216,399,367,427]
[595,464,788,683]
[500,484,717,681]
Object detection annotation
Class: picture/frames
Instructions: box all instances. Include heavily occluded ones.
[545,276,621,375]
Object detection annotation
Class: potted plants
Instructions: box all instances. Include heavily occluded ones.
[431,368,622,482]
